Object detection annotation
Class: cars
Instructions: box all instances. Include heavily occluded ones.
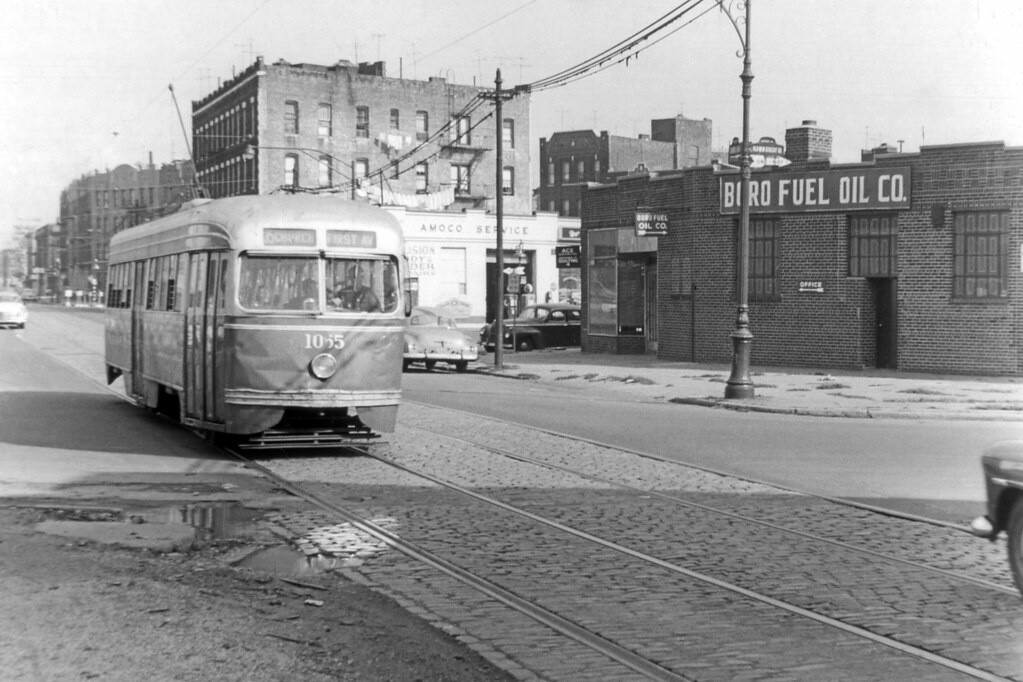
[404,304,479,371]
[0,291,25,330]
[481,304,583,350]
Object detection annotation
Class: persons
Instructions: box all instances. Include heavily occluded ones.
[332,265,382,313]
[253,285,270,308]
[284,279,337,309]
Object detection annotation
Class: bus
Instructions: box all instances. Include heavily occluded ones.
[105,193,406,450]
